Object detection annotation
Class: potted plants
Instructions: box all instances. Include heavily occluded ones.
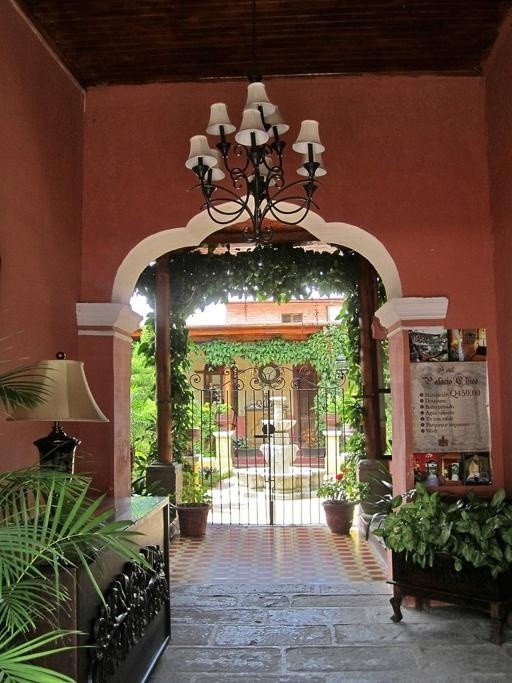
[365,481,512,643]
[173,484,218,538]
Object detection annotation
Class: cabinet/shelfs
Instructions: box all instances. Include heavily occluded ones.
[1,495,170,682]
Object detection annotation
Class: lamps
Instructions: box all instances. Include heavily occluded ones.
[6,349,108,478]
[185,1,328,244]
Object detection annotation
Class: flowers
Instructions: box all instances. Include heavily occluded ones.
[317,454,370,502]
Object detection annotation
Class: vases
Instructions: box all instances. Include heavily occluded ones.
[323,500,356,533]
